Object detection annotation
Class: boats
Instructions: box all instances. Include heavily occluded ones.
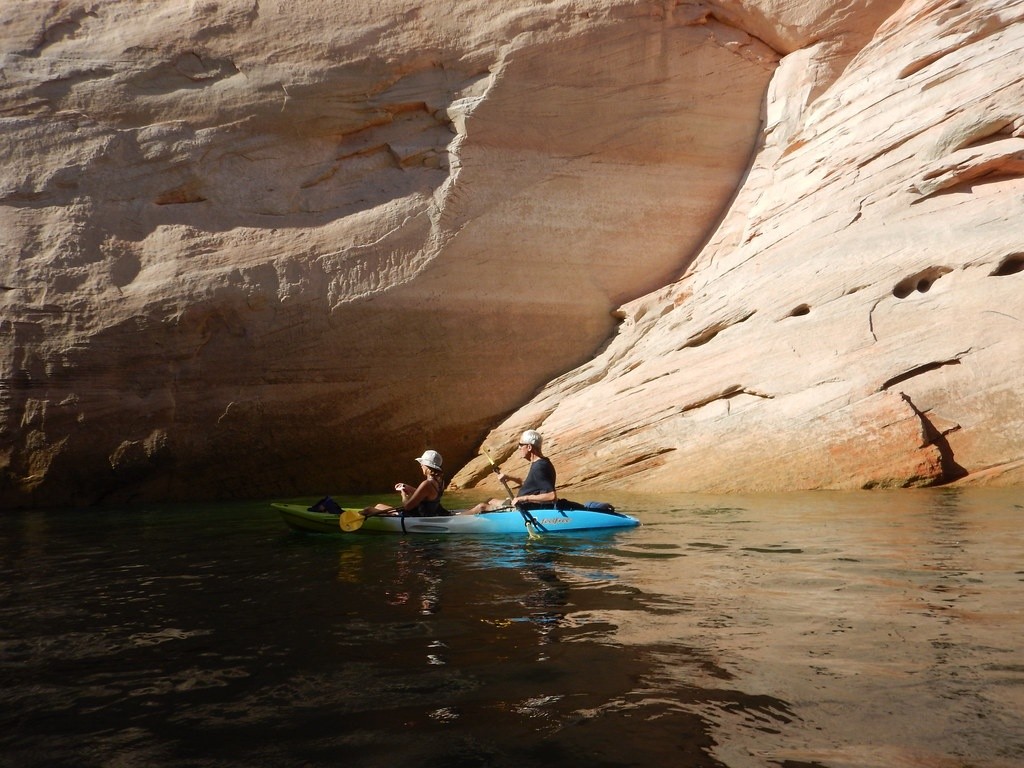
[268,500,642,532]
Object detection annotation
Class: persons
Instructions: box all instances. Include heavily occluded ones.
[358,450,446,517]
[460,429,556,515]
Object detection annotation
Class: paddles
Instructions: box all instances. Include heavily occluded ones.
[481,446,538,539]
[339,504,403,530]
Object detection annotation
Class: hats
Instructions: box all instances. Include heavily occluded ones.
[415,449,443,472]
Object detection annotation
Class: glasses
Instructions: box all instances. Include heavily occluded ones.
[519,442,535,447]
